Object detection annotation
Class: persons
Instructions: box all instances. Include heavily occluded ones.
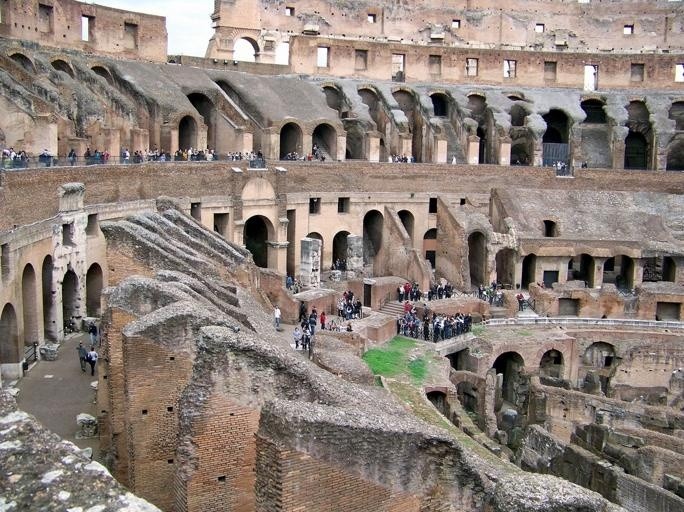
[89,320,97,345]
[293,301,328,349]
[286,271,303,294]
[2,148,29,168]
[388,153,414,164]
[40,147,111,165]
[76,340,98,376]
[551,158,570,176]
[281,144,325,162]
[228,149,266,164]
[397,281,473,343]
[123,147,218,164]
[274,304,282,328]
[479,280,503,307]
[337,290,362,320]
[516,293,524,312]
